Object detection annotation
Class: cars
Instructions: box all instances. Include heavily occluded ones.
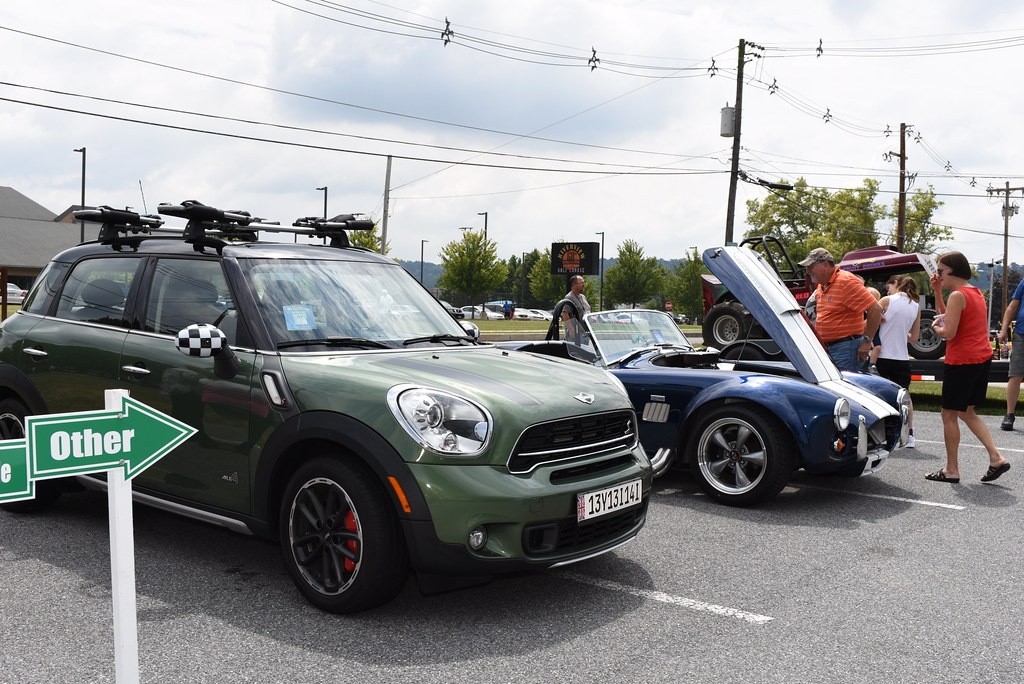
[489,246,907,504]
[392,305,424,320]
[530,309,562,321]
[0,282,29,305]
[591,313,650,326]
[461,306,505,320]
[667,312,690,323]
[512,308,545,320]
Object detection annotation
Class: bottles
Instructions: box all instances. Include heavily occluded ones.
[990,336,1009,359]
[918,291,925,308]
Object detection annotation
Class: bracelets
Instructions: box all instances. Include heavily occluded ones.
[932,324,938,330]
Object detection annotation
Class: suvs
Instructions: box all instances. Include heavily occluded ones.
[0,198,655,617]
[438,300,466,318]
[698,233,948,359]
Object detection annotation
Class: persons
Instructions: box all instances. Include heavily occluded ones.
[503,301,515,320]
[560,275,591,345]
[796,248,883,373]
[998,278,1024,431]
[806,275,921,448]
[924,251,1010,483]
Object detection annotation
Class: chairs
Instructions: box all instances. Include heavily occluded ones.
[65,279,126,325]
[161,279,222,332]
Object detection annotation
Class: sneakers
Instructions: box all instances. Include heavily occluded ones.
[1000,413,1015,431]
[905,435,914,448]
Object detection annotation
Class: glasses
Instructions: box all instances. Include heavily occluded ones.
[937,267,950,277]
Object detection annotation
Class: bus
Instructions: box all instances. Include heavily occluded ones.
[485,300,517,313]
[479,304,504,313]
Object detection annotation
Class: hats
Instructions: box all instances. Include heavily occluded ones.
[797,247,835,266]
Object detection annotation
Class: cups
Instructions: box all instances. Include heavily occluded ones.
[933,314,948,341]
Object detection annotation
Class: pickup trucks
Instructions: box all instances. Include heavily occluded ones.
[989,336,1013,350]
[113,281,131,298]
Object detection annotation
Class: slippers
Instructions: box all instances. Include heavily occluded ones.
[981,463,1010,481]
[924,469,960,483]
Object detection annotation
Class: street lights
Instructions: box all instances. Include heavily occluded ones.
[594,232,606,310]
[478,212,488,255]
[459,227,474,261]
[72,147,87,243]
[316,187,327,245]
[988,256,1004,336]
[521,252,528,309]
[421,240,430,283]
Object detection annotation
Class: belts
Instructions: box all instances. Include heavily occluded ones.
[824,334,863,348]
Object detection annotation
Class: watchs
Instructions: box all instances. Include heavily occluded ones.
[864,336,873,341]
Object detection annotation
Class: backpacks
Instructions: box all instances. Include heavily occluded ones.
[511,305,515,312]
